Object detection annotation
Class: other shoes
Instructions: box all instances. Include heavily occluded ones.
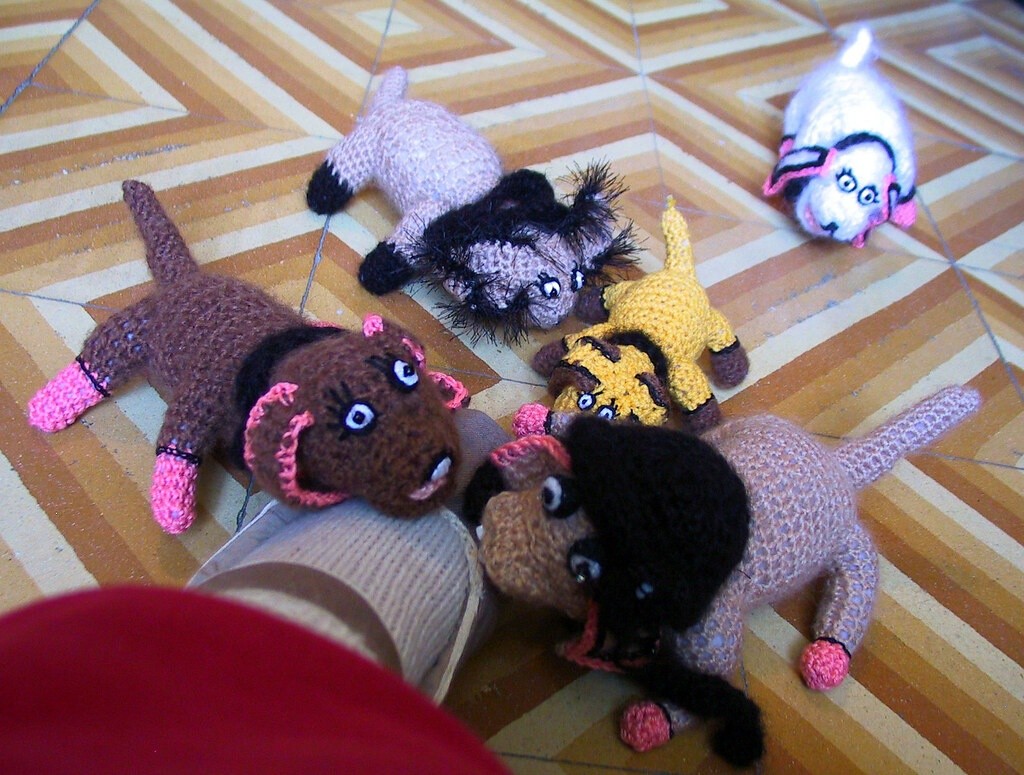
[186,406,513,706]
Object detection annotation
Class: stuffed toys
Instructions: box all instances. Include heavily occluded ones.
[26,180,472,536]
[461,382,985,770]
[761,24,919,251]
[530,194,751,436]
[306,66,651,347]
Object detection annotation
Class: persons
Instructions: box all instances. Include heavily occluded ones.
[0,405,513,775]
